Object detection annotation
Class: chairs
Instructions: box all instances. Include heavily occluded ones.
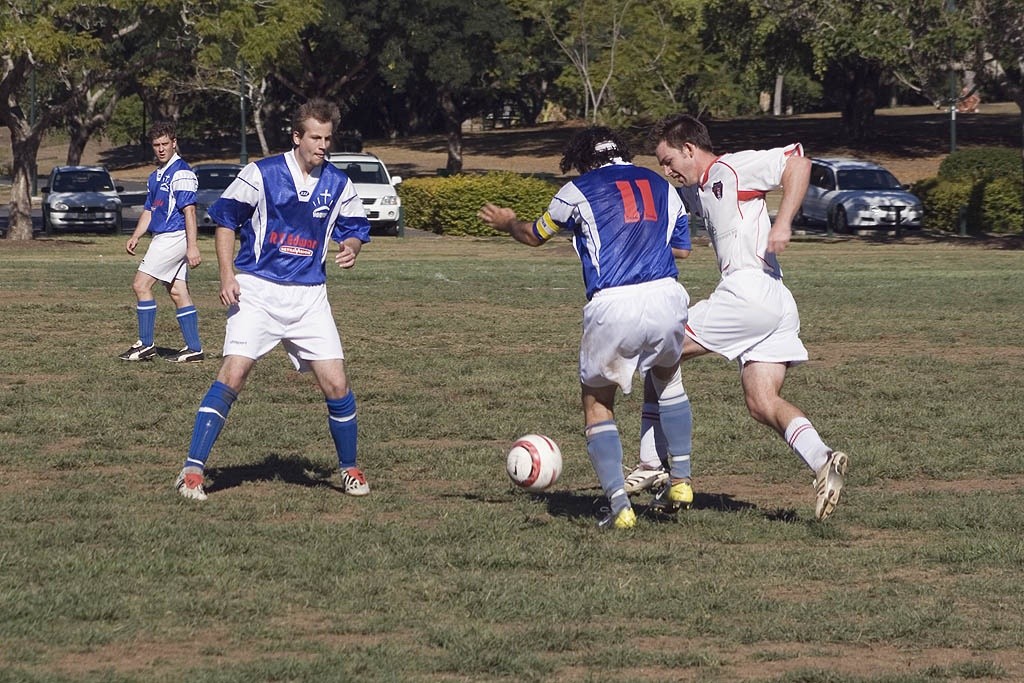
[347,163,361,181]
[59,176,104,189]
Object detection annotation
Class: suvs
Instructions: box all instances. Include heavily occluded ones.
[791,155,925,233]
[322,150,403,235]
[40,164,125,237]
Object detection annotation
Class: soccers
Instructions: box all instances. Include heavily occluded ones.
[507,435,563,489]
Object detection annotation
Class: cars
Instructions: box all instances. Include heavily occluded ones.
[189,163,250,234]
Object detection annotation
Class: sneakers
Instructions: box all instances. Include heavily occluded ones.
[649,483,693,514]
[175,472,208,500]
[341,469,370,496]
[813,451,848,522]
[625,465,671,497]
[598,506,637,528]
[164,345,204,364]
[118,340,157,360]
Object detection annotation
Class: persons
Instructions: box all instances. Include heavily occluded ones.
[173,97,371,502]
[118,122,206,364]
[478,125,693,530]
[622,112,851,525]
[955,88,981,114]
